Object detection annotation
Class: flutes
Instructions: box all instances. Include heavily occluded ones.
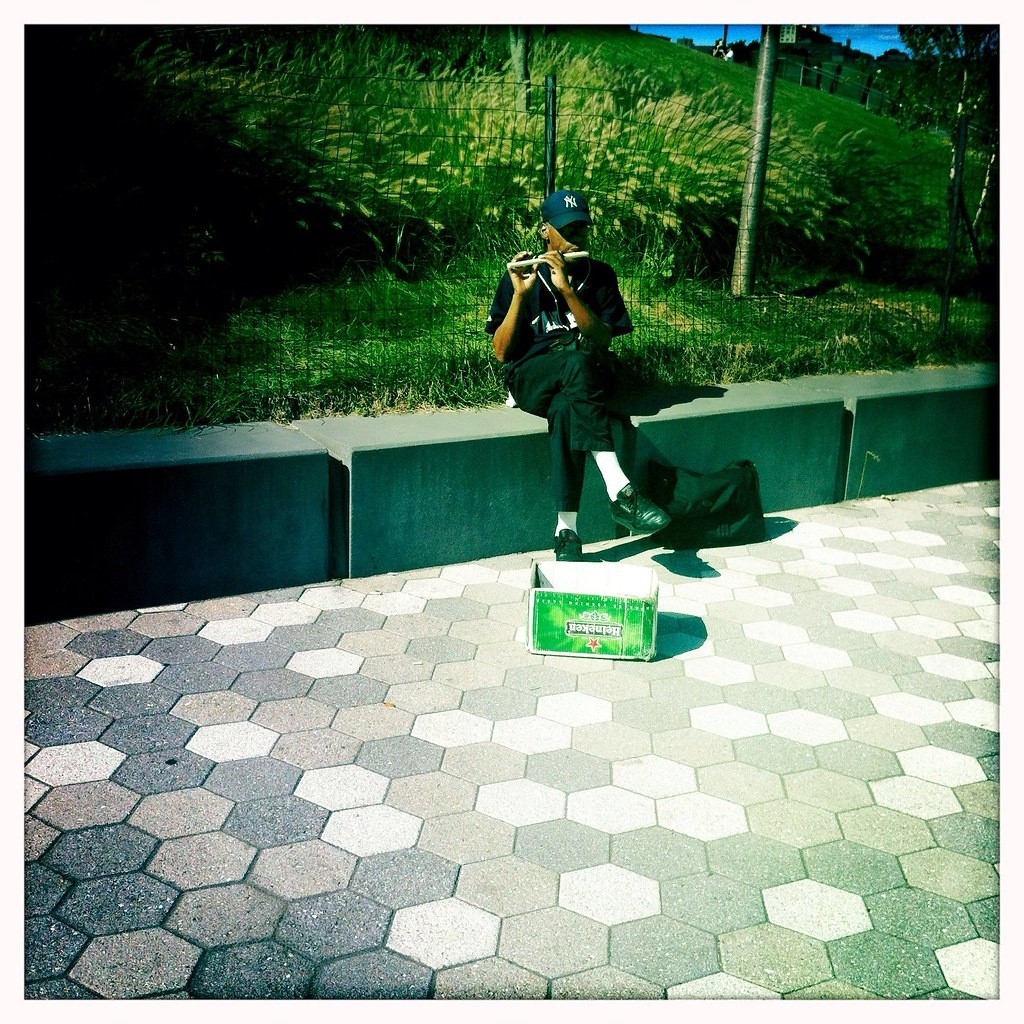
[506,250,590,267]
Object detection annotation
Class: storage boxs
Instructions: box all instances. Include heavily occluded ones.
[524,558,660,661]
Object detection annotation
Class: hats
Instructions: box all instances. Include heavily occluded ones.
[542,190,593,230]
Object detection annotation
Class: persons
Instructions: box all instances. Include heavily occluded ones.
[482,189,674,563]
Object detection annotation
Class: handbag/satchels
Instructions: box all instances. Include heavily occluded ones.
[640,459,765,550]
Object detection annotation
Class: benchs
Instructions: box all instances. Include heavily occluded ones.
[24,362,999,627]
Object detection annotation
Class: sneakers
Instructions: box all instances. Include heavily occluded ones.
[610,482,671,534]
[554,529,582,561]
